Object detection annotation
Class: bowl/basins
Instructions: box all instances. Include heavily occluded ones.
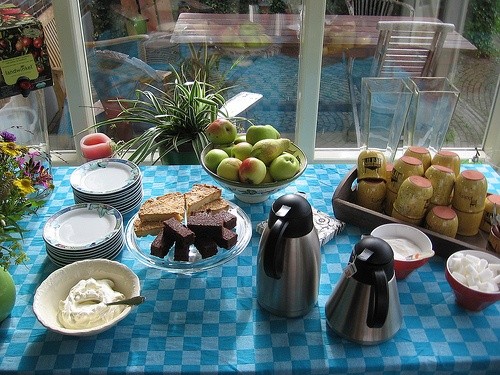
[216,46,267,67]
[201,134,307,204]
[445,250,500,310]
[32,259,140,337]
[369,223,435,278]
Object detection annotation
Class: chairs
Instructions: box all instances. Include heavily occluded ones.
[38,5,173,121]
[344,0,454,148]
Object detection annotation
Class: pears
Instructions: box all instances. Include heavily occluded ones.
[249,138,294,165]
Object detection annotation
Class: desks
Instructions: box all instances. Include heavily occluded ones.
[170,12,477,50]
[0,164,500,375]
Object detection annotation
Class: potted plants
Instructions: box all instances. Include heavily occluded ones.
[71,62,252,165]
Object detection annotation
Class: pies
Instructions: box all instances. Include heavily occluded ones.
[134,185,229,237]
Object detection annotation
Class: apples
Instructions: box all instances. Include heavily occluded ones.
[205,119,300,185]
[220,22,275,47]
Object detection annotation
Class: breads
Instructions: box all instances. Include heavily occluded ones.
[150,210,239,262]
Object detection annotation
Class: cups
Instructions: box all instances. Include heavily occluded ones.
[356,145,500,257]
[320,20,372,57]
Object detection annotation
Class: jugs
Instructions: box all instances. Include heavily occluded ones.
[324,234,402,345]
[255,192,320,317]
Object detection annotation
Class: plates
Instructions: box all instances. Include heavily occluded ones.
[123,197,253,274]
[143,40,180,64]
[70,158,143,216]
[42,202,125,268]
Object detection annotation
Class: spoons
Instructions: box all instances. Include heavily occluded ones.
[80,296,146,306]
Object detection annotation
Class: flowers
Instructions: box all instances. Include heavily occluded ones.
[0,126,69,272]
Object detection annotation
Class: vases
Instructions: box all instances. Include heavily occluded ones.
[0,265,16,322]
